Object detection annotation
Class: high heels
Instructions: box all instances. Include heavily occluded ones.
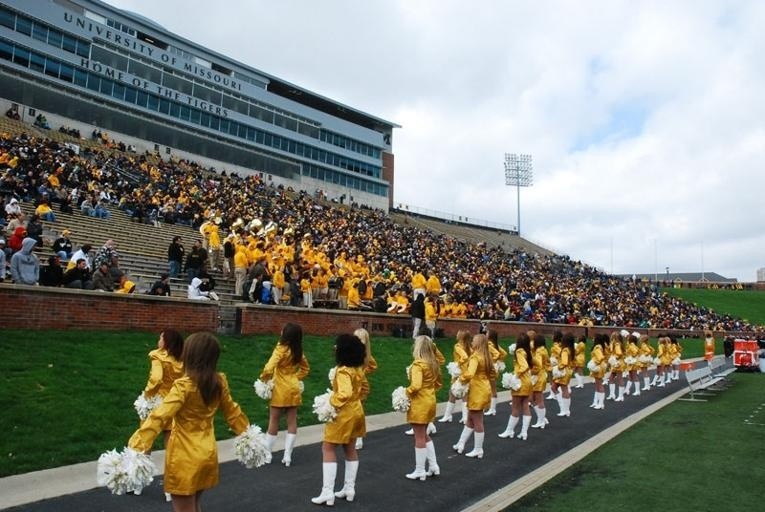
[405,426,416,436]
[426,422,437,437]
[354,437,365,450]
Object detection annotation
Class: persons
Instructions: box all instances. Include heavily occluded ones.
[1,106,765,512]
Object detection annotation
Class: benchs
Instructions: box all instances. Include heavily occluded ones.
[1,197,244,308]
[0,114,128,155]
[277,187,375,217]
[708,354,738,387]
[678,366,728,402]
[387,210,555,256]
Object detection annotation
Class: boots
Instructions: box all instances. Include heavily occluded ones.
[547,391,556,400]
[672,369,680,380]
[651,373,658,386]
[465,431,485,460]
[659,376,666,388]
[606,383,616,401]
[615,386,624,403]
[625,380,633,396]
[452,425,473,455]
[602,374,609,386]
[425,441,441,478]
[643,377,651,391]
[439,400,455,423]
[459,400,469,424]
[311,460,338,508]
[484,396,497,416]
[594,392,606,410]
[574,373,585,389]
[666,372,671,383]
[532,406,550,429]
[336,460,360,502]
[405,448,427,482]
[518,413,532,441]
[589,392,599,407]
[555,394,572,416]
[261,432,277,467]
[498,413,519,439]
[634,381,641,397]
[281,433,296,466]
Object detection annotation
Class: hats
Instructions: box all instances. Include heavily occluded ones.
[62,229,72,234]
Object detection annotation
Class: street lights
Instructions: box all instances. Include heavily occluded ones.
[504,152,534,236]
